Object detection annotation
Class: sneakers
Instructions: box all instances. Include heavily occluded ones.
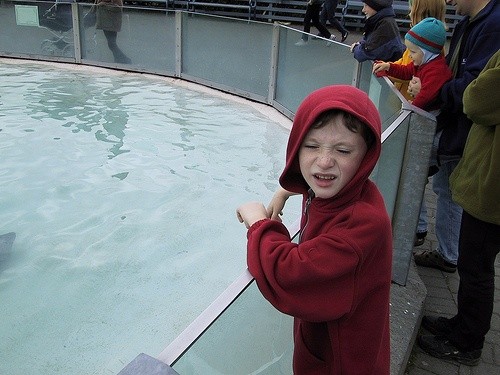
[327,34,336,47]
[414,236,426,246]
[422,316,485,349]
[414,250,456,273]
[311,33,324,40]
[418,335,482,366]
[295,39,308,48]
[341,31,350,43]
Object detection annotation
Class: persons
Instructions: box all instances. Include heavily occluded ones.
[294,0,500,230]
[236,85,392,375]
[416,51,500,365]
[95,0,131,65]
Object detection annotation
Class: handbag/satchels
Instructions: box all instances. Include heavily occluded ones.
[83,11,97,28]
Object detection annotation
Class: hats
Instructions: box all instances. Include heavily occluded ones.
[360,0,393,12]
[405,18,446,54]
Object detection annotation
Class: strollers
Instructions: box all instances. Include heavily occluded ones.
[34,1,88,59]
